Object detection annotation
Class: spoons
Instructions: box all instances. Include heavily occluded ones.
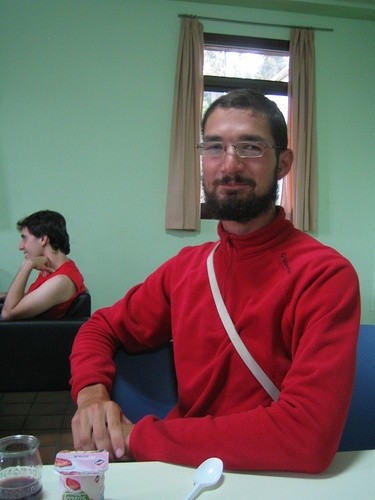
[184,456,224,500]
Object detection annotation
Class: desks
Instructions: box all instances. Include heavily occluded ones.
[0,451,375,500]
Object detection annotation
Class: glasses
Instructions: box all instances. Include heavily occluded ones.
[194,141,281,159]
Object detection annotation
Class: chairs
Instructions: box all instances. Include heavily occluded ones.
[115,323,375,462]
[0,291,91,393]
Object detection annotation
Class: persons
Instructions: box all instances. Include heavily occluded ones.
[0,209,88,320]
[69,87,362,476]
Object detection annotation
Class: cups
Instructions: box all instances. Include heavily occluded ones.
[0,435,45,500]
